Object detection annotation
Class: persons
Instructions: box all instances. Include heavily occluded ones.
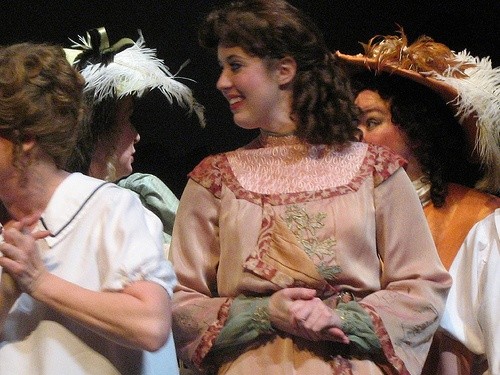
[0,43,181,374]
[167,0,452,375]
[61,28,205,233]
[334,24,500,375]
[436,209,500,375]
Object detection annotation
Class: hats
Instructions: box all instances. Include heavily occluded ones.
[334,42,500,186]
[64,47,139,97]
